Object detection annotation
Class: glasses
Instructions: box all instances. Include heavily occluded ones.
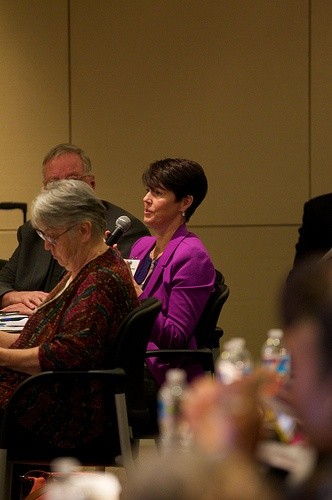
[45,175,87,186]
[36,222,79,243]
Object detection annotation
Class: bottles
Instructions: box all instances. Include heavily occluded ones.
[158,369,191,454]
[218,337,253,386]
[260,327,290,374]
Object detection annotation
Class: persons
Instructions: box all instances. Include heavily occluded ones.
[182,260,332,500]
[0,143,151,315]
[289,192,332,281]
[0,180,140,449]
[105,158,217,382]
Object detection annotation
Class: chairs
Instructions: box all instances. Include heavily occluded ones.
[0,270,230,500]
